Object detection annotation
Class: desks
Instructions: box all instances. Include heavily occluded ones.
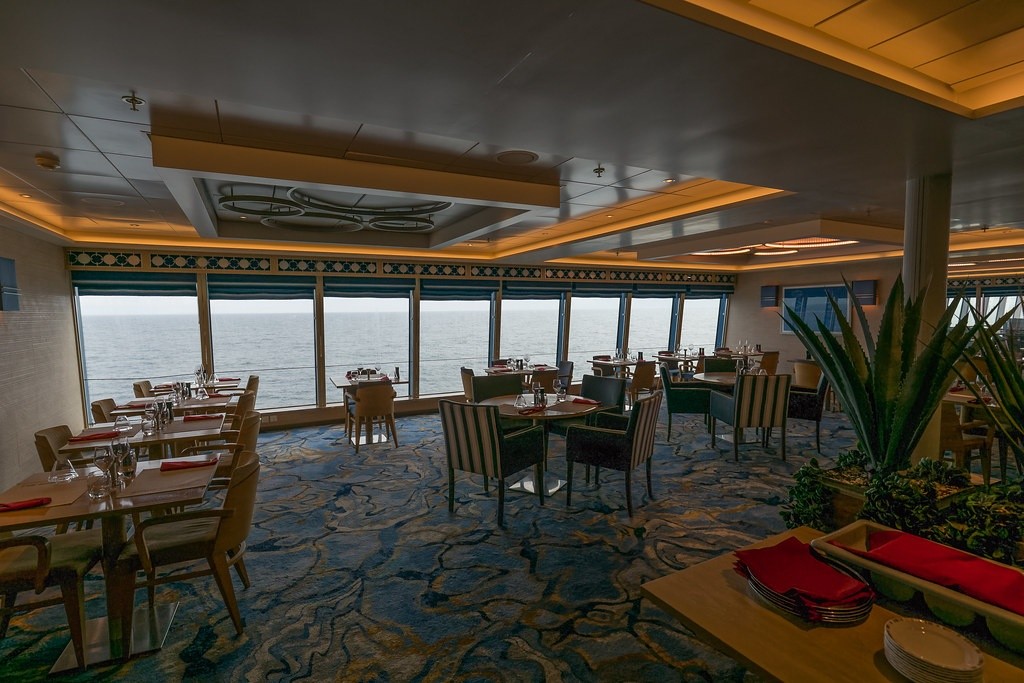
[636,524,1024,683]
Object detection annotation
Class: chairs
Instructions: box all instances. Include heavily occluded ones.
[0,371,265,676]
[330,337,1024,531]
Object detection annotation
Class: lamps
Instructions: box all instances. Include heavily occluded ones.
[760,285,778,307]
[853,279,876,305]
[0,257,22,312]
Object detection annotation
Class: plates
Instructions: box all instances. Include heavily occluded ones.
[747,550,874,623]
[884,618,984,683]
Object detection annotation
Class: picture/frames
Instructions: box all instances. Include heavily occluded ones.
[782,283,851,335]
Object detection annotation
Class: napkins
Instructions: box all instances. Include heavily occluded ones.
[730,527,1024,631]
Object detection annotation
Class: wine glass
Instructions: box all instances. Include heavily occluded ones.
[524,353,531,371]
[553,378,561,404]
[532,381,542,408]
[617,347,632,363]
[93,436,130,492]
[168,365,207,406]
[674,344,698,357]
[375,362,381,379]
[737,358,755,374]
[145,396,166,433]
[975,375,991,396]
[358,363,363,379]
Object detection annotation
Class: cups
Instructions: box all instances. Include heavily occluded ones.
[119,451,137,478]
[196,387,209,401]
[141,419,156,436]
[47,458,79,485]
[208,374,219,386]
[351,371,358,381]
[557,388,566,402]
[86,470,112,498]
[514,395,527,409]
[113,415,133,432]
